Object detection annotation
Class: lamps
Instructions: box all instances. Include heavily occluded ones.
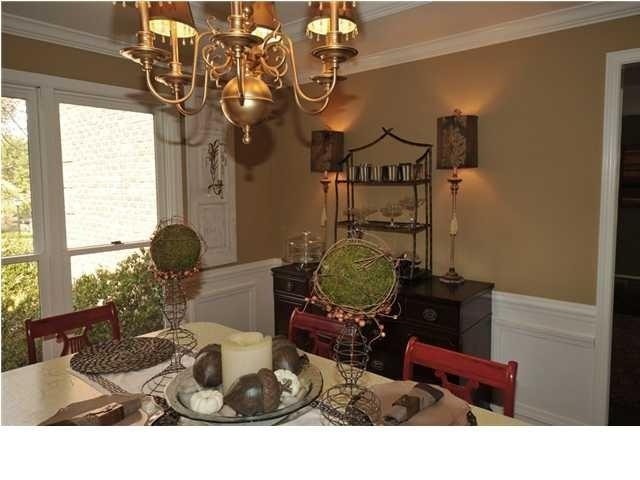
[436,108,479,285]
[109,1,359,145]
[309,129,345,259]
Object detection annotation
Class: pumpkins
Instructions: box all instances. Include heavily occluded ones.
[190,390,224,415]
[274,369,299,397]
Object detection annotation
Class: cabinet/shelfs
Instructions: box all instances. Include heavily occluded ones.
[334,126,434,284]
[270,262,495,410]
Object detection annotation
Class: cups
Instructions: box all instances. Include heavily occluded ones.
[348,162,424,182]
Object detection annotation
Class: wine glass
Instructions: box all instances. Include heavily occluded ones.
[398,198,425,229]
[343,207,378,225]
[380,207,403,228]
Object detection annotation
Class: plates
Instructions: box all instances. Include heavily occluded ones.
[163,362,324,423]
[37,392,180,426]
[345,380,478,425]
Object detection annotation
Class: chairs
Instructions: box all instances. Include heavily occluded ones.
[403,338,517,418]
[25,303,121,364]
